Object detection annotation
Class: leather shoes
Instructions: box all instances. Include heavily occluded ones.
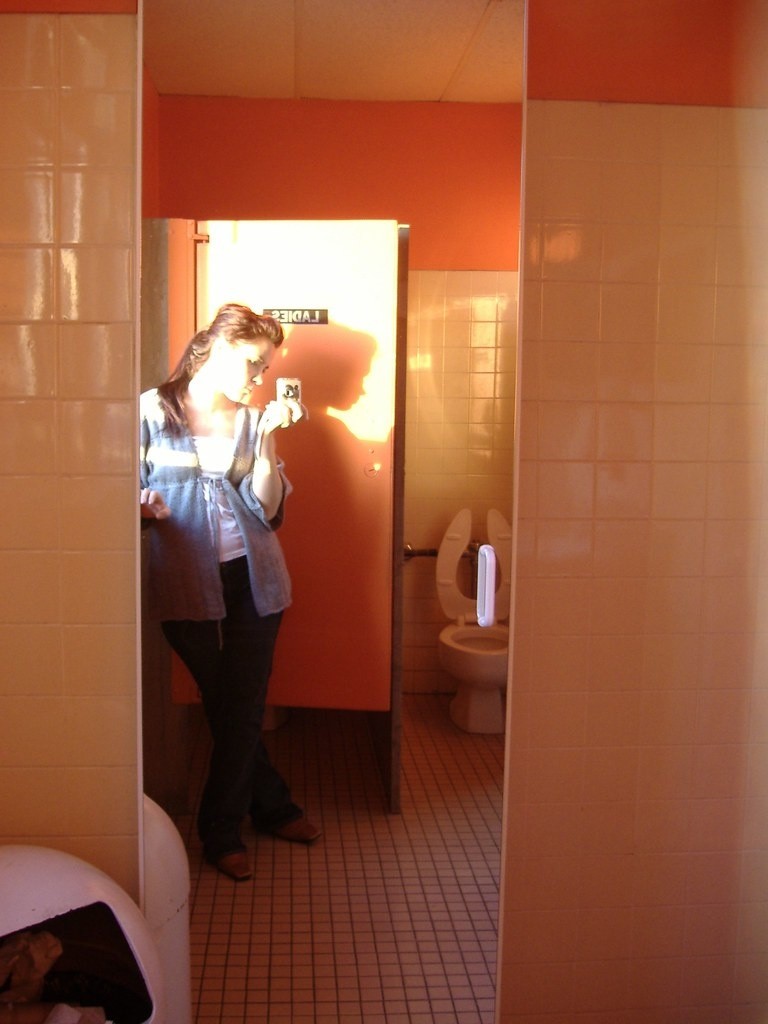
[213,851,253,882]
[275,819,322,844]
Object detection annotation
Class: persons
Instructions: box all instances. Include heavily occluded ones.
[140,303,323,880]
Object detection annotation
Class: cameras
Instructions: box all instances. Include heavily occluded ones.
[276,377,302,415]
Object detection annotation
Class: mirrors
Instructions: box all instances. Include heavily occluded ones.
[137,0,528,1024]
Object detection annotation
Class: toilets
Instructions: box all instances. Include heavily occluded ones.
[435,508,513,735]
